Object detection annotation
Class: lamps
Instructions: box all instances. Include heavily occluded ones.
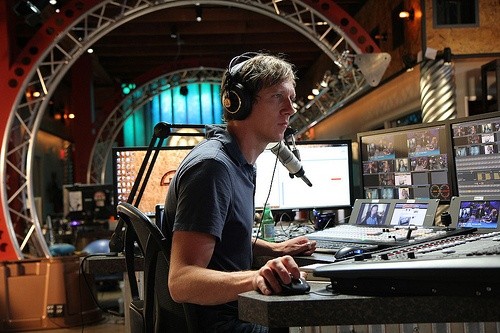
[48,0,60,11]
[32,79,41,98]
[398,8,414,21]
[320,70,334,88]
[307,93,316,100]
[170,24,177,38]
[334,50,350,68]
[195,7,202,22]
[50,107,62,120]
[311,78,323,96]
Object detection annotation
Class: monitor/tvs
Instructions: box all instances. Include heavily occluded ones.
[450,110,500,202]
[357,118,456,203]
[253,139,356,211]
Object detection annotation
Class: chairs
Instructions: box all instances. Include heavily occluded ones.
[117,202,241,333]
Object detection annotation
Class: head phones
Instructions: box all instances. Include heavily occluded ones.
[222,51,258,115]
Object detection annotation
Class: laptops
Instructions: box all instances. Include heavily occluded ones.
[255,270,311,296]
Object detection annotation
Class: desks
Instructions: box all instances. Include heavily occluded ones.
[238,254,500,333]
[78,255,144,333]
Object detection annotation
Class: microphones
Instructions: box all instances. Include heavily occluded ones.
[269,142,313,187]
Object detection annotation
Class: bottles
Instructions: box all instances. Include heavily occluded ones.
[260,203,275,243]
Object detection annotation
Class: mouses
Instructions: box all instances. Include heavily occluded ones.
[335,246,367,259]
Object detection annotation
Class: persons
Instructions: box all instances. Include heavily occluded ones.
[154,51,316,333]
